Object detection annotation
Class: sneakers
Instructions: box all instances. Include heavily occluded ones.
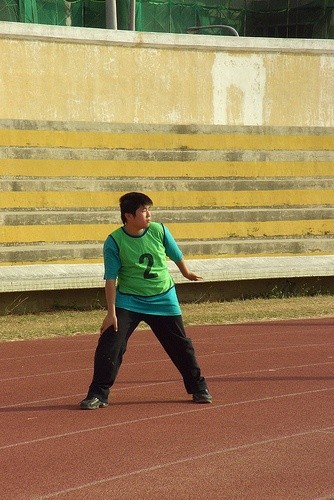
[78,395,109,409]
[193,388,213,404]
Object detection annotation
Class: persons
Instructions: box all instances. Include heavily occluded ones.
[79,191,212,411]
